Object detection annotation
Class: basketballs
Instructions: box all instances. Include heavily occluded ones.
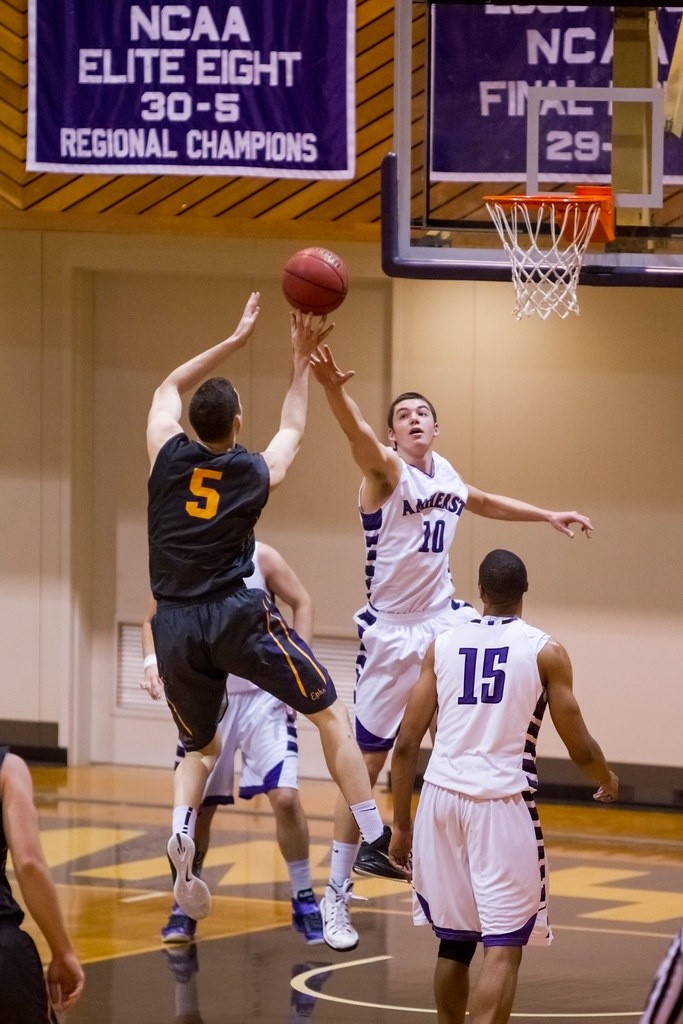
[280,244,351,318]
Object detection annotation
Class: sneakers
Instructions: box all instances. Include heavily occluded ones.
[291,962,332,1018]
[160,902,196,944]
[291,888,325,945]
[353,826,413,883]
[164,945,196,983]
[165,832,212,920]
[318,878,360,951]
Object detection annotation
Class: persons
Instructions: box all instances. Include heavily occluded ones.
[0,746,86,1024]
[145,290,413,922]
[388,547,621,1024]
[139,541,330,948]
[305,343,596,953]
